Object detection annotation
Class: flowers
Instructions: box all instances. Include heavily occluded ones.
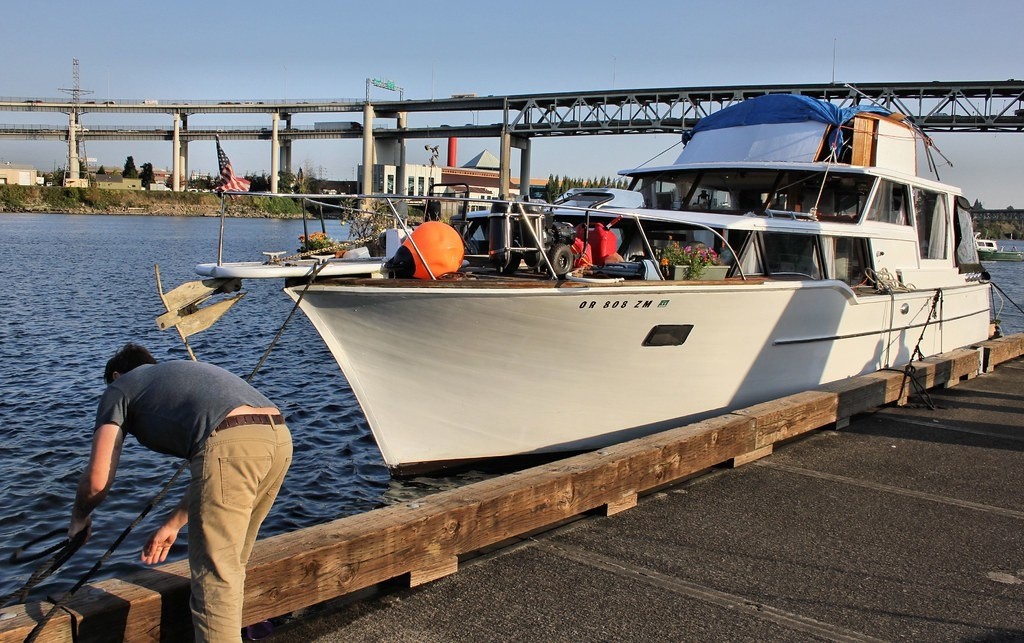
[340,219,345,226]
[296,232,340,254]
[655,235,725,281]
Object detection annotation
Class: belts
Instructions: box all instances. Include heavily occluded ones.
[213,414,286,432]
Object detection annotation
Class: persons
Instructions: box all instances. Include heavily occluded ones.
[68,341,294,643]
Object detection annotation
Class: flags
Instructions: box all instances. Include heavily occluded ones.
[211,136,251,202]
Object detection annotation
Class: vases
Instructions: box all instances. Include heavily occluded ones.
[669,263,732,281]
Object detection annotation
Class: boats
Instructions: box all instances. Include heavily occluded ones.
[196,90,996,482]
